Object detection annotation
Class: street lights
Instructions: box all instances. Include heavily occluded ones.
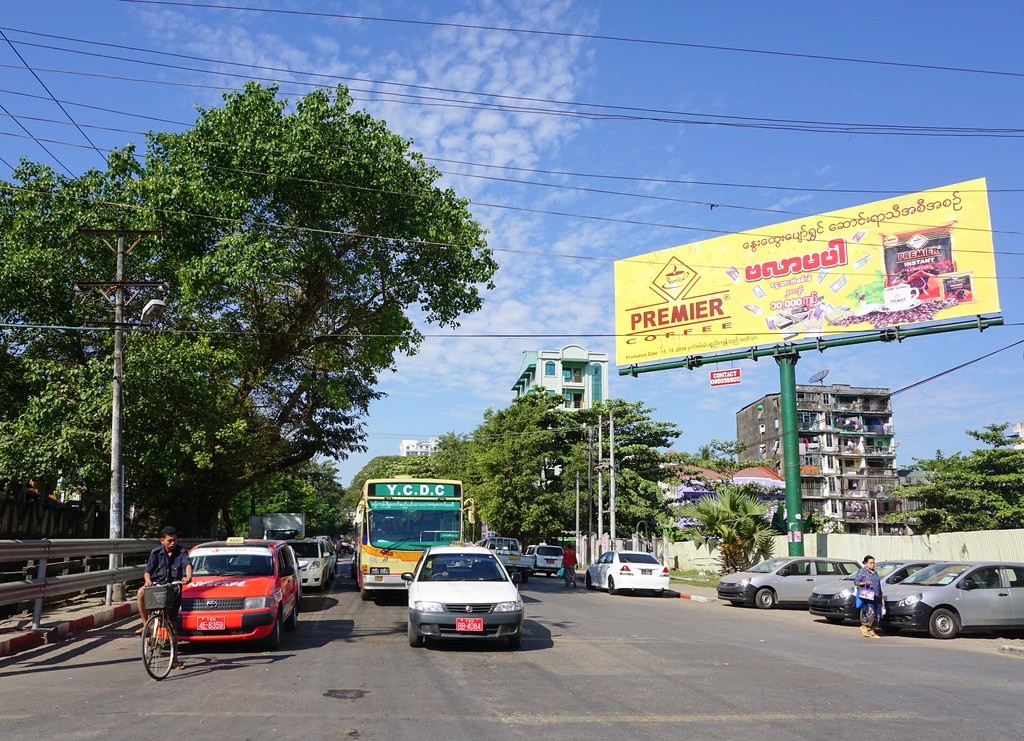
[109,298,167,606]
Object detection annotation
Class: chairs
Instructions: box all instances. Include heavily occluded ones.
[250,555,267,573]
[206,557,226,573]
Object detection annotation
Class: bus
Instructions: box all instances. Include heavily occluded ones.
[355,474,476,600]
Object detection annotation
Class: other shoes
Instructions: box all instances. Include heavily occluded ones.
[564,586,569,588]
[170,663,185,670]
[135,626,151,634]
[859,625,869,637]
[574,585,577,588]
[868,630,881,639]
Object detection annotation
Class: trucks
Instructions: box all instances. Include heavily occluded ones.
[479,536,535,583]
[248,512,306,541]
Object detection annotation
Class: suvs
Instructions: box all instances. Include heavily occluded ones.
[158,539,301,651]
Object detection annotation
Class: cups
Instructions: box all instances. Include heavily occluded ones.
[883,285,919,311]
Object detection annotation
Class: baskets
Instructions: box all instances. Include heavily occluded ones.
[143,586,178,613]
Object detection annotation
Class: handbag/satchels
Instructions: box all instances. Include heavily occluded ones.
[855,582,875,608]
[556,564,565,580]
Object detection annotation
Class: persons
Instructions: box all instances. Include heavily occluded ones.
[853,555,886,639]
[424,562,452,579]
[561,543,578,588]
[197,556,226,572]
[134,526,194,670]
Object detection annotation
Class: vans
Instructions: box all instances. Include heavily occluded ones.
[879,561,1024,639]
[525,544,564,576]
[716,555,863,610]
[808,559,950,627]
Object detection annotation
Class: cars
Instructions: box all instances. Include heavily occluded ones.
[283,539,338,592]
[401,545,525,650]
[351,554,355,578]
[586,549,670,597]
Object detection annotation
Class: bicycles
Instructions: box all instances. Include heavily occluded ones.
[140,580,188,680]
